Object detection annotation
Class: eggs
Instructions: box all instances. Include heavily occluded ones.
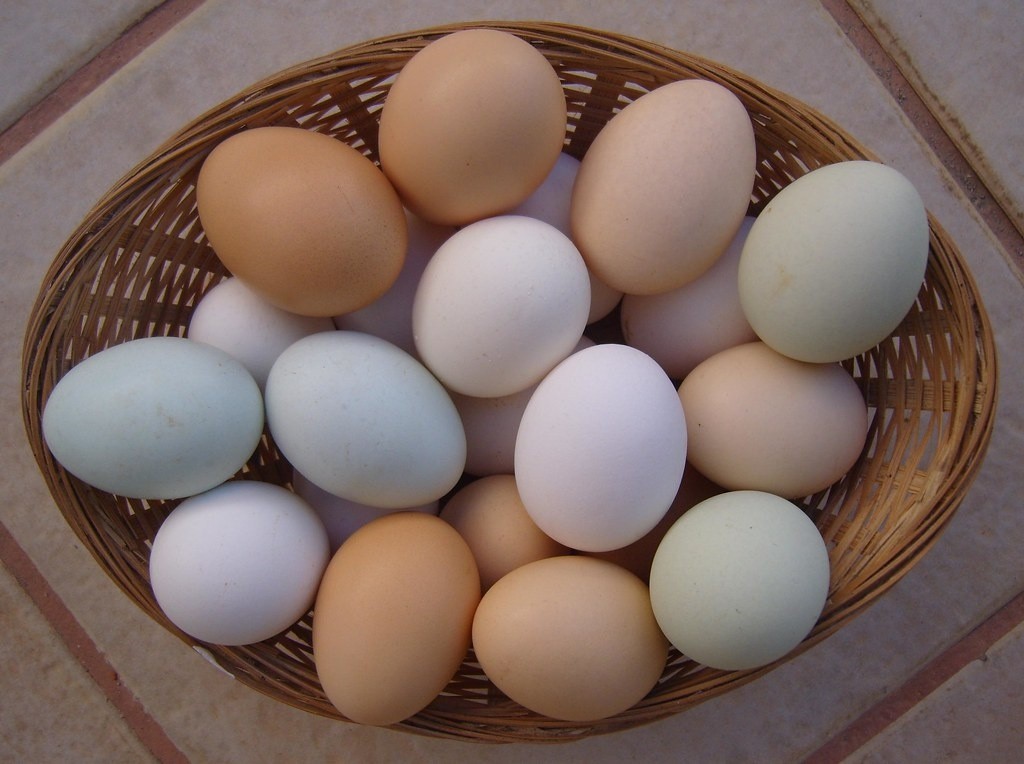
[39,29,929,727]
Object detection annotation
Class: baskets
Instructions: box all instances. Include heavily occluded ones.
[21,21,999,742]
